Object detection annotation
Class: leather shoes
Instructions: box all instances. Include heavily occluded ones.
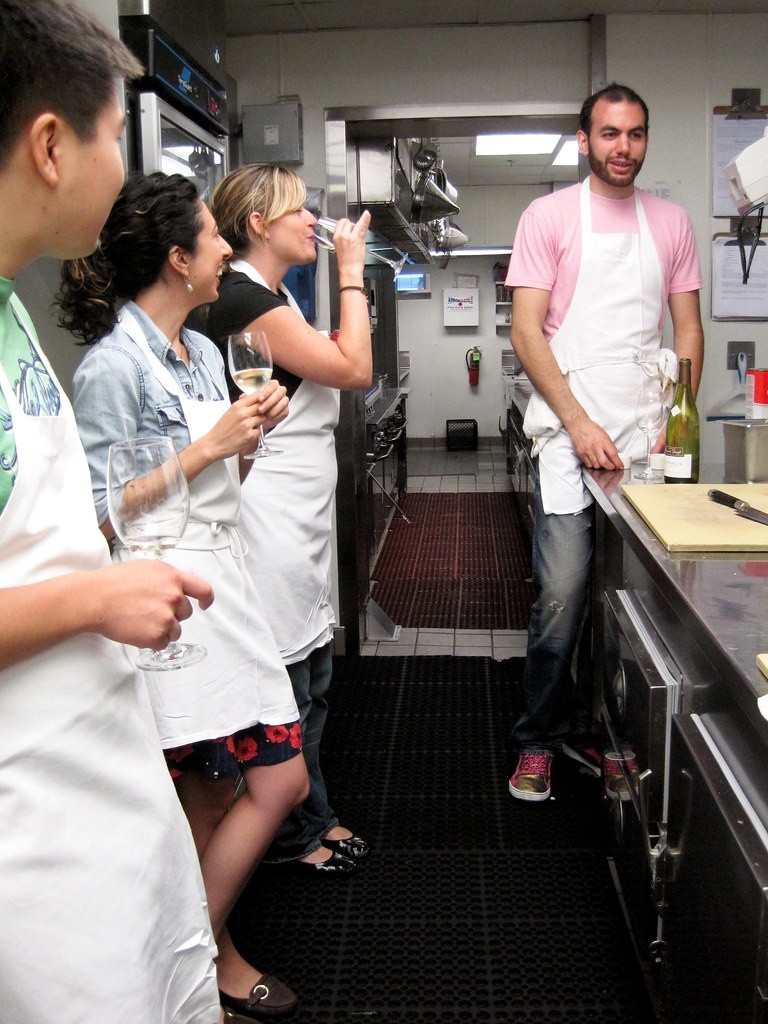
[290,846,358,880]
[320,833,372,860]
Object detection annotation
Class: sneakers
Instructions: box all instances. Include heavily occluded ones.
[509,755,551,801]
[561,742,603,777]
[604,745,640,802]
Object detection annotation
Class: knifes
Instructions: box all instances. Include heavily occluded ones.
[708,489,768,525]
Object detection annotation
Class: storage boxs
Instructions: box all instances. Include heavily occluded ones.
[493,269,508,281]
[446,419,478,450]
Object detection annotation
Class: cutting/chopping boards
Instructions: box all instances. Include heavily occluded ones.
[620,483,768,552]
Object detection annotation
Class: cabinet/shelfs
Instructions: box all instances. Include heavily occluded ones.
[494,281,513,326]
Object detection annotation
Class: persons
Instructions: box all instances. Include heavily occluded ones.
[502,81,710,800]
[0,0,260,1024]
[67,177,297,1011]
[199,163,372,875]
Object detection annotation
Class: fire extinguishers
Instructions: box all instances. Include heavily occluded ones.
[465,346,482,385]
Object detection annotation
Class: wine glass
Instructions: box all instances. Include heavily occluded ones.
[634,389,662,480]
[313,216,408,282]
[106,437,207,670]
[227,331,283,460]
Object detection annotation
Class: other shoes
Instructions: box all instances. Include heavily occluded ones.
[223,1005,263,1024]
[218,973,298,1014]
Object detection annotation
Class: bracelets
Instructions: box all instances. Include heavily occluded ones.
[339,285,369,297]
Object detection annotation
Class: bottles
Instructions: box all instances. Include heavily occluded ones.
[664,358,699,483]
